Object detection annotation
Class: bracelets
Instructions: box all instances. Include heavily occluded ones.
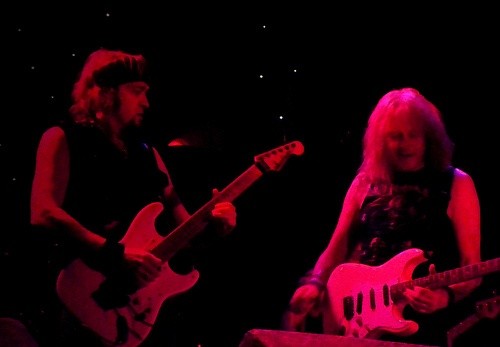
[439,287,456,312]
[301,269,327,292]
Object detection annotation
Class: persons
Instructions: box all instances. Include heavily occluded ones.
[289,87,482,347]
[30,50,237,347]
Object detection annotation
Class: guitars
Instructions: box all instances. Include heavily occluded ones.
[322,246,499,339]
[55,140,306,347]
[446,296,499,341]
